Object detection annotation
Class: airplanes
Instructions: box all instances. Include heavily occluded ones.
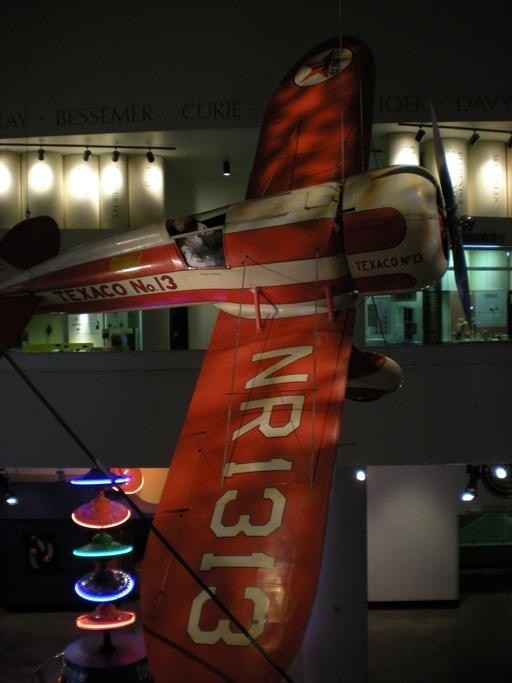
[1,35,474,683]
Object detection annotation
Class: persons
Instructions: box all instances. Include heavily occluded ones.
[171,213,226,268]
[455,317,468,341]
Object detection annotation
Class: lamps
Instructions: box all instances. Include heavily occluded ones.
[72,469,144,628]
[222,160,231,175]
[395,122,512,149]
[3,143,175,164]
